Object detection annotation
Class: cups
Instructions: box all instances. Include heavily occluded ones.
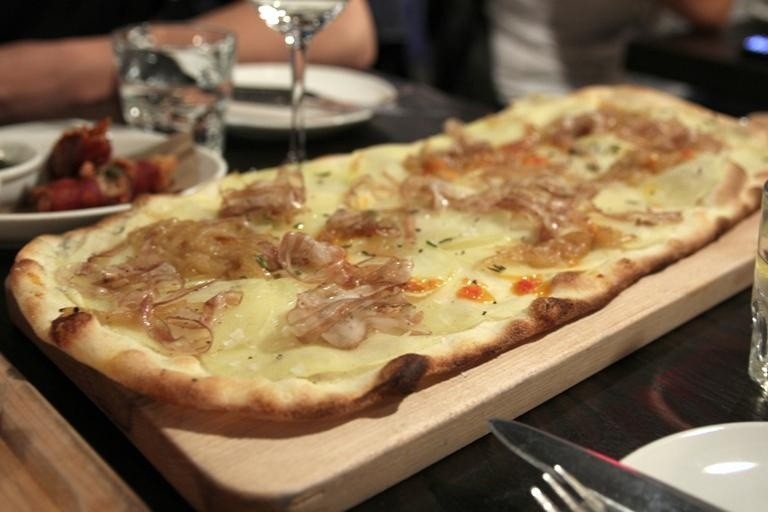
[108,21,237,155]
[745,179,767,390]
[0,137,43,200]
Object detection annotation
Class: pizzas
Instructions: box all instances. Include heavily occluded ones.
[5,83,768,424]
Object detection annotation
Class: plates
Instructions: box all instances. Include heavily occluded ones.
[223,56,398,137]
[622,419,768,512]
[0,120,225,241]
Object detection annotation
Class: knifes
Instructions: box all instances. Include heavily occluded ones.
[232,81,382,120]
[487,411,727,511]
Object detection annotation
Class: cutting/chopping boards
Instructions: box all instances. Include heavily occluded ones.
[3,84,766,511]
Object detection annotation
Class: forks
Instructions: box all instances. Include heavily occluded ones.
[530,464,616,512]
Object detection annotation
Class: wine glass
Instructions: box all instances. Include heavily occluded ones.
[255,0,355,168]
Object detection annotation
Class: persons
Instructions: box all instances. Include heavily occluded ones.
[0,0,419,128]
